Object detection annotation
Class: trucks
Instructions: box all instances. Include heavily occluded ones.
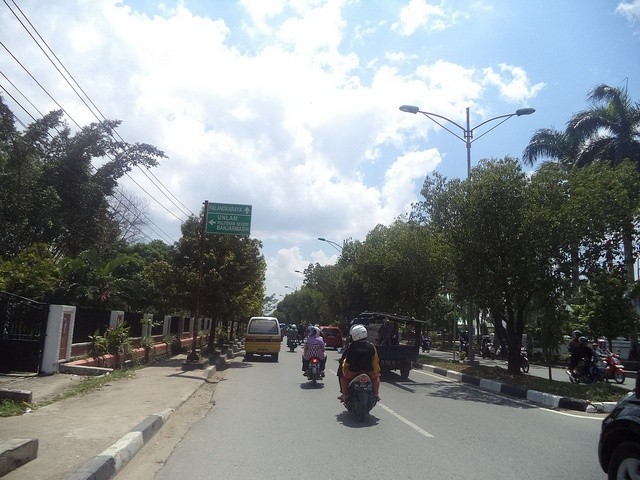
[347,311,430,382]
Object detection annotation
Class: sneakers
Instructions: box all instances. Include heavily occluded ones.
[374,394,381,401]
[337,395,352,403]
[303,371,309,376]
[321,370,325,378]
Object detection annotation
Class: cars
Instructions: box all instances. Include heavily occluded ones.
[598,387,639,480]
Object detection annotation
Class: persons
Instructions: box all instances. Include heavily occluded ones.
[596,339,613,368]
[285,323,300,346]
[592,343,598,363]
[577,336,597,367]
[458,330,470,351]
[481,335,490,358]
[340,324,384,406]
[569,330,584,363]
[421,330,429,347]
[301,327,327,378]
[297,321,319,342]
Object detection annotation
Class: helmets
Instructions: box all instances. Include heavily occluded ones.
[308,326,314,336]
[349,324,358,345]
[572,330,582,339]
[351,325,368,342]
[578,337,590,345]
[310,326,321,336]
[291,325,297,329]
[598,339,607,348]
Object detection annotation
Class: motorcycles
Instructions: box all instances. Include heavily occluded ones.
[459,339,468,358]
[300,342,327,386]
[286,338,300,351]
[479,341,496,360]
[335,347,381,421]
[519,347,530,374]
[420,337,431,353]
[597,353,625,384]
[566,356,611,385]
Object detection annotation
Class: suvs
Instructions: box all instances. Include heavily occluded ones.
[319,325,343,350]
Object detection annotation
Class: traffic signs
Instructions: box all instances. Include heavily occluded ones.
[205,212,252,236]
[208,203,252,216]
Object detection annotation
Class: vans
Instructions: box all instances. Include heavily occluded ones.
[245,317,281,363]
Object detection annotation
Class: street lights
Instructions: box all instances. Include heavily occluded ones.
[318,237,345,257]
[279,294,285,299]
[284,285,298,293]
[398,102,537,369]
[294,269,309,281]
